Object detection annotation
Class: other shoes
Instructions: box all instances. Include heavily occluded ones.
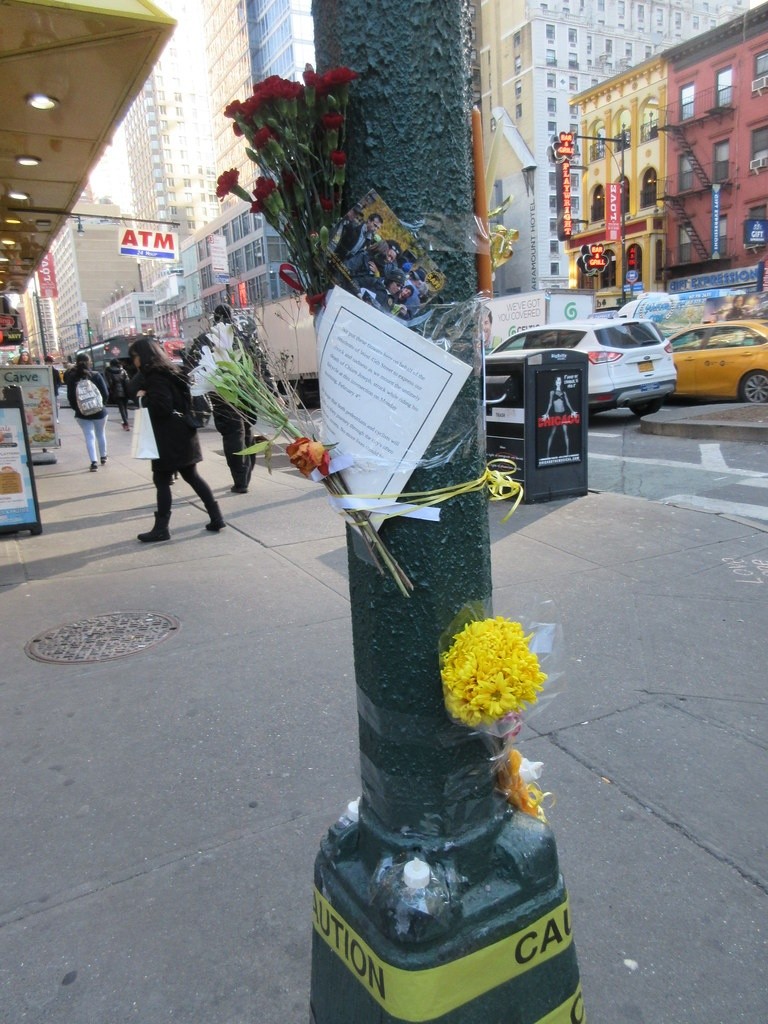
[90,461,98,472]
[231,486,247,493]
[122,422,130,431]
[101,457,107,465]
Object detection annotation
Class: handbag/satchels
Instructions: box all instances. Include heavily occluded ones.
[131,396,159,459]
[123,371,133,400]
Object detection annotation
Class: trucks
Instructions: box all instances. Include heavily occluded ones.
[486,288,598,351]
[254,291,320,409]
[178,304,255,357]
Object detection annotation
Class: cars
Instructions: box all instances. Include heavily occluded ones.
[666,318,768,405]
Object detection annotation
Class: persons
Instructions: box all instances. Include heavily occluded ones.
[110,343,123,357]
[683,330,705,350]
[541,374,581,458]
[482,305,504,350]
[332,210,426,319]
[180,304,285,493]
[725,294,748,319]
[68,353,109,473]
[106,358,130,431]
[18,351,68,424]
[127,338,226,542]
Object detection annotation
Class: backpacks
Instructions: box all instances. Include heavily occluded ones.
[173,365,212,428]
[75,376,103,416]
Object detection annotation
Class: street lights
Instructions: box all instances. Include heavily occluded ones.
[119,316,138,330]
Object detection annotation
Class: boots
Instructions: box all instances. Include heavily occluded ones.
[138,511,170,541]
[204,501,226,531]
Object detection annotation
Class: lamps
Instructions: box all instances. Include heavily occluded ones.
[67,215,85,237]
[24,94,61,112]
[15,155,41,166]
[8,192,31,200]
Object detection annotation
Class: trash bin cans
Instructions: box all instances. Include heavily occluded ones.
[486,350,589,506]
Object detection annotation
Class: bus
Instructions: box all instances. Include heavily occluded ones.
[75,333,165,403]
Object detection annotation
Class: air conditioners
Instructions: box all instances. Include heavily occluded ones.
[749,156,768,176]
[751,75,768,96]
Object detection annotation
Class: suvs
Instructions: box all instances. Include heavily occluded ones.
[486,317,679,418]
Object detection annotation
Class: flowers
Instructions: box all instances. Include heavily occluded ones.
[436,594,570,823]
[165,307,416,596]
[214,54,364,315]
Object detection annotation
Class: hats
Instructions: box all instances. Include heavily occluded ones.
[214,303,232,321]
